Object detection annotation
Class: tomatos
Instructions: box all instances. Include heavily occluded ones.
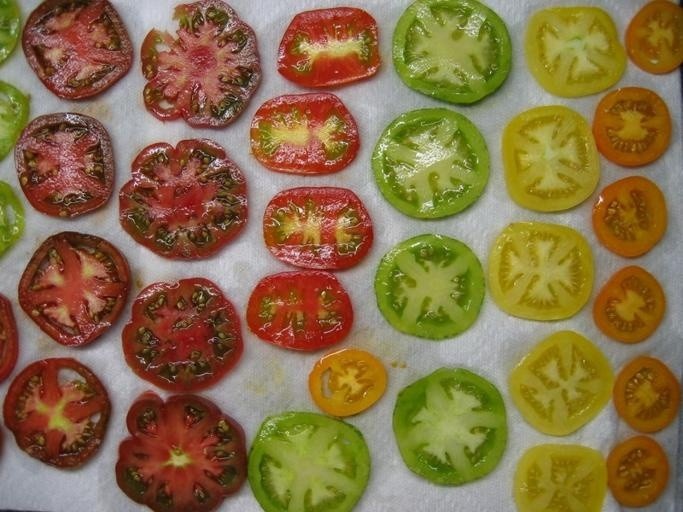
[1,1,682,512]
